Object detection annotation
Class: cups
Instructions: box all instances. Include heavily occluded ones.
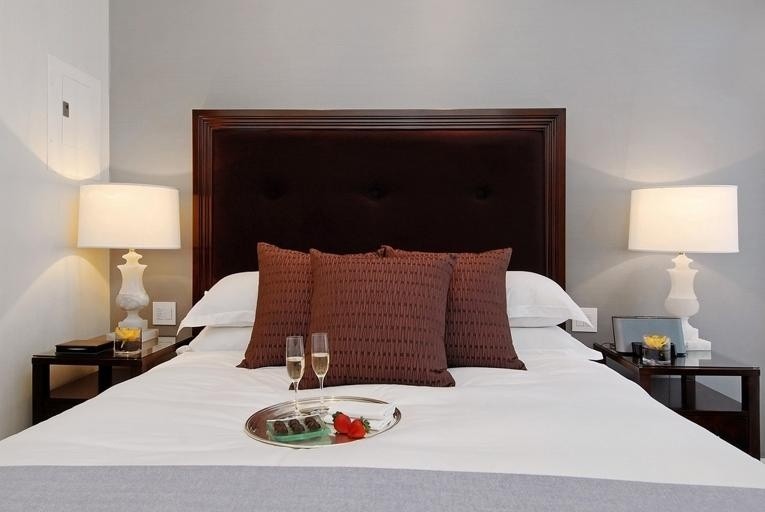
[642,335,672,366]
[114,325,144,356]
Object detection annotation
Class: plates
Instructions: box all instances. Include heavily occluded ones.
[245,395,402,449]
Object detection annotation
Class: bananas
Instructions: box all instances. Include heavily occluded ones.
[115,327,141,340]
[644,335,669,349]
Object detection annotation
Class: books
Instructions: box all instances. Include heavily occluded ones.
[55,346,113,357]
[55,339,113,353]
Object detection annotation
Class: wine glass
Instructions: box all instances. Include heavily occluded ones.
[308,332,332,409]
[283,335,307,414]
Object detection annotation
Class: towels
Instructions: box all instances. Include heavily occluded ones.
[318,398,396,431]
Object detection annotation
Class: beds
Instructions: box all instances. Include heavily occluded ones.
[0,107,765,511]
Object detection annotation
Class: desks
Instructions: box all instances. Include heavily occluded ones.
[25,333,188,428]
[594,338,763,460]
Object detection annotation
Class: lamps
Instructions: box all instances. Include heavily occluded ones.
[72,179,186,345]
[625,177,743,360]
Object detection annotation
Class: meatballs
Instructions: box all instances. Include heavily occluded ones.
[275,417,321,435]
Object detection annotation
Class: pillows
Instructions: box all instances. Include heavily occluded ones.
[498,268,590,330]
[235,241,320,370]
[288,247,463,392]
[178,266,254,331]
[445,248,527,375]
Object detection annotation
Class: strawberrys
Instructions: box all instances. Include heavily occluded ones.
[334,432,352,443]
[348,415,372,438]
[332,411,351,434]
